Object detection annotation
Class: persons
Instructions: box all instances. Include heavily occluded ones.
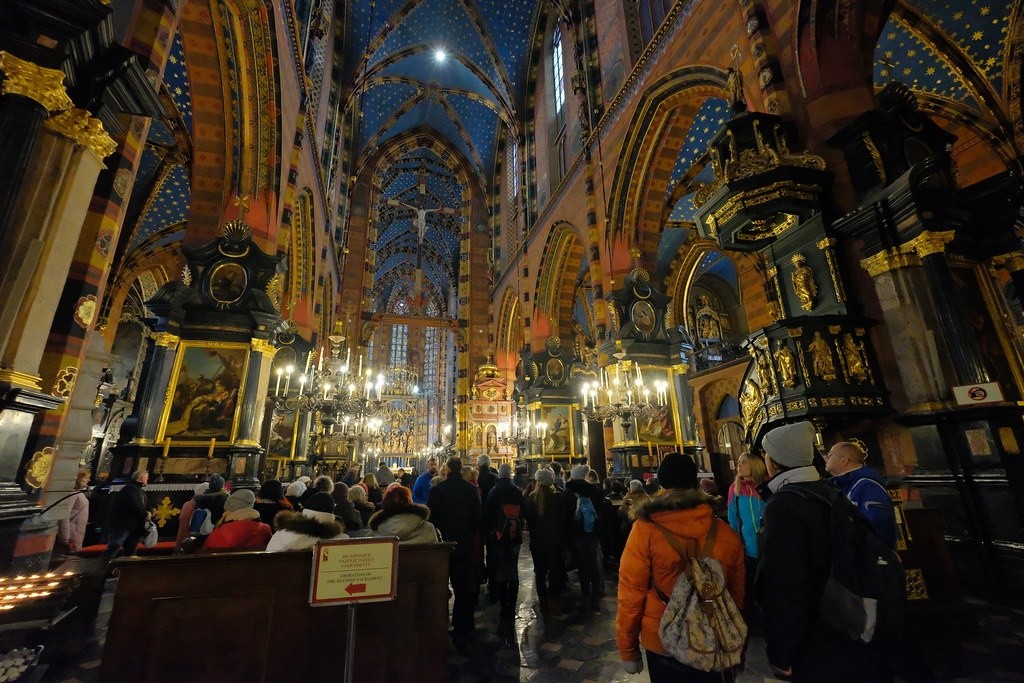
[844,333,862,376]
[728,452,771,560]
[523,461,658,607]
[743,378,762,427]
[774,340,797,380]
[823,442,898,549]
[752,421,882,683]
[756,349,770,388]
[809,332,835,375]
[791,254,814,305]
[615,452,746,683]
[55,468,91,554]
[375,454,522,633]
[99,469,152,566]
[175,464,438,554]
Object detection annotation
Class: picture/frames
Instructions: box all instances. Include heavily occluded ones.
[153,339,252,445]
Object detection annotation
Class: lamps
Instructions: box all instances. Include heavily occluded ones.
[270,347,444,458]
[580,364,670,431]
[496,411,549,453]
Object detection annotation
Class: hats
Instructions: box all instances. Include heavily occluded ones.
[224,489,255,512]
[762,422,816,468]
[298,476,312,485]
[700,478,717,493]
[209,474,225,491]
[630,480,643,491]
[286,481,307,497]
[535,469,554,486]
[570,465,590,479]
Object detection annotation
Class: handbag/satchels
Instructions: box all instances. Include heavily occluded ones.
[139,519,158,548]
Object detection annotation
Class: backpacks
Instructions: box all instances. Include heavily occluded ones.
[652,515,749,672]
[573,491,597,532]
[783,484,907,654]
[494,486,523,545]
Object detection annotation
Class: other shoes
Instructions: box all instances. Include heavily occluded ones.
[450,587,604,657]
